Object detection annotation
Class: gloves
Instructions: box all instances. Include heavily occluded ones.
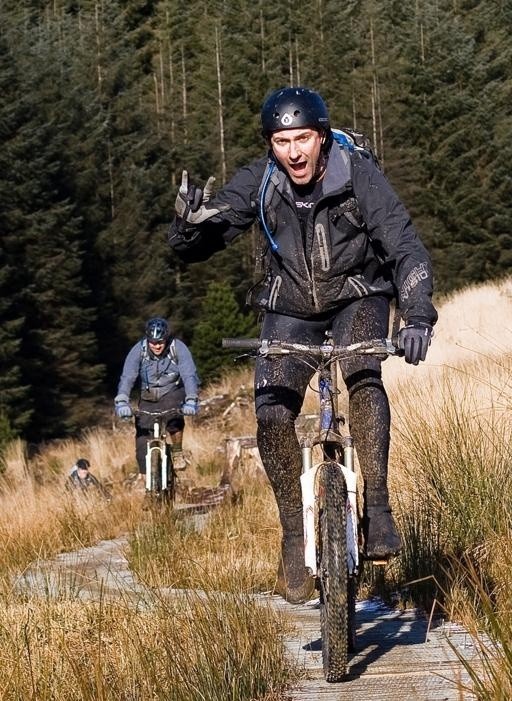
[397,318,431,365]
[172,170,231,242]
[115,400,132,418]
[182,399,197,416]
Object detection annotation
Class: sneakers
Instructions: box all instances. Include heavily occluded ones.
[172,443,186,470]
[279,530,315,605]
[361,507,402,558]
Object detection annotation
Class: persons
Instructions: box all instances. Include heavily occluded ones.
[113,317,204,493]
[163,86,441,610]
[63,458,111,499]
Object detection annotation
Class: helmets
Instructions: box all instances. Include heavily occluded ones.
[143,318,170,342]
[261,88,330,142]
[76,459,90,470]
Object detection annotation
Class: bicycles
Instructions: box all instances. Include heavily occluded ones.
[218,332,429,683]
[126,404,187,528]
